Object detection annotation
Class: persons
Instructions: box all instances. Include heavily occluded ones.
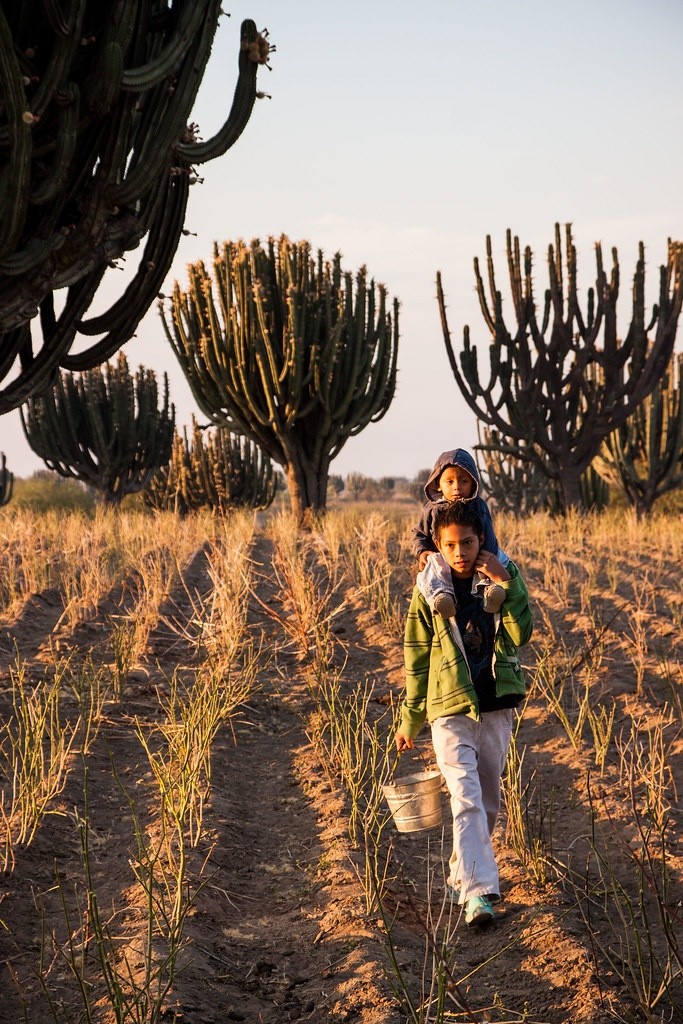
[393,502,535,926]
[412,448,507,620]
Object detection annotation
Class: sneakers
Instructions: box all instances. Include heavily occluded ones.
[464,895,496,928]
[448,886,462,905]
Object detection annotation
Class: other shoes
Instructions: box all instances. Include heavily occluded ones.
[482,584,506,614]
[433,592,457,620]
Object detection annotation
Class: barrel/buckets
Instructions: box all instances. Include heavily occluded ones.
[382,772,442,832]
